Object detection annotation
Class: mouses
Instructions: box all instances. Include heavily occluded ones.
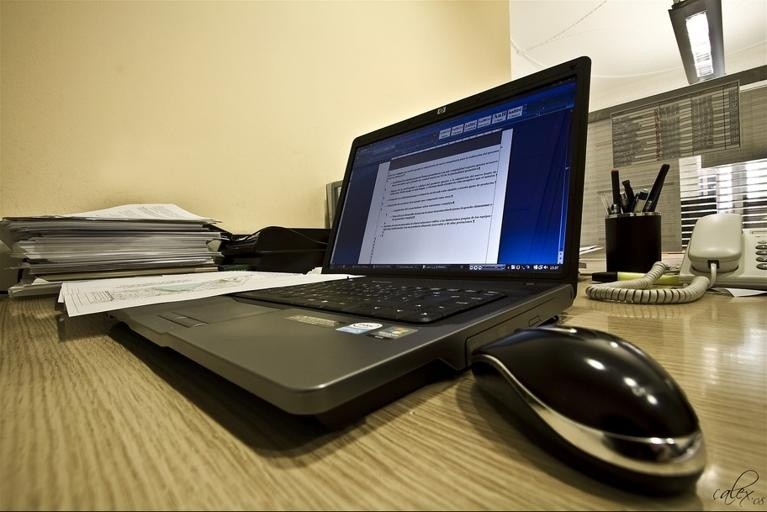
[468,320,708,499]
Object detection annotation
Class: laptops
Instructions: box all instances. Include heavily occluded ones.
[107,56,592,415]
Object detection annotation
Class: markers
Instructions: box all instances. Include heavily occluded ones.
[642,163,670,212]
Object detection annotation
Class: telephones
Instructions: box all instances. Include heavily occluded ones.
[585,213,767,305]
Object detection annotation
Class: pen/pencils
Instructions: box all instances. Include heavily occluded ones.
[600,167,648,214]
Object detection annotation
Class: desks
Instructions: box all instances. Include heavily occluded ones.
[0,284,762,510]
